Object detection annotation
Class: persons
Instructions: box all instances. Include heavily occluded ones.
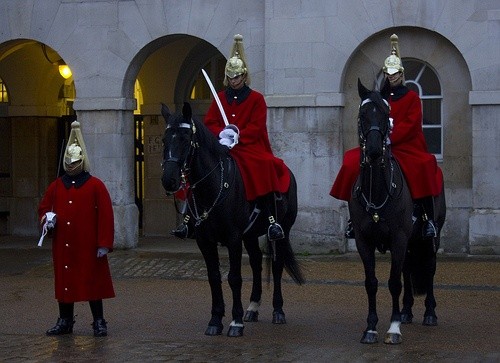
[171,34,291,240]
[329,34,443,238]
[37,120,114,336]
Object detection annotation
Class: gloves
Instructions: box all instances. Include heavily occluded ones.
[97,248,110,258]
[219,128,236,146]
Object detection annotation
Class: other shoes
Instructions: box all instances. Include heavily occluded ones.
[168,224,193,237]
[423,218,440,237]
[46,317,76,335]
[267,222,282,238]
[345,223,354,240]
[93,319,108,337]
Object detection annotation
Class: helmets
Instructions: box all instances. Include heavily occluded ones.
[383,34,405,86]
[63,120,91,173]
[222,33,250,87]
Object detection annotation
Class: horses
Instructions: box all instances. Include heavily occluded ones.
[345,75,449,346]
[158,99,309,339]
